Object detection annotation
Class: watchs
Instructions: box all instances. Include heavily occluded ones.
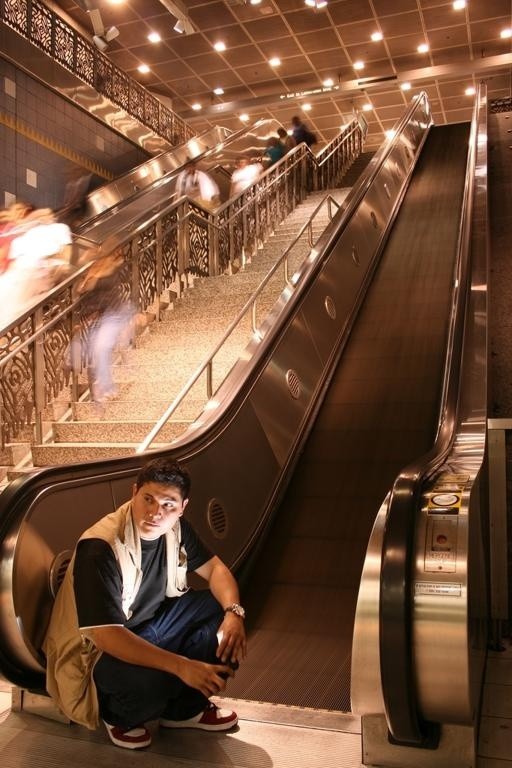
[220,602,247,619]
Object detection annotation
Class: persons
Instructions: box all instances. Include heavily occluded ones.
[0,198,34,279]
[0,203,74,332]
[70,235,148,409]
[170,114,317,213]
[41,456,249,752]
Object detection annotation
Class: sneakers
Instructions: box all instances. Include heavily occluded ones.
[158,700,239,733]
[104,718,153,749]
